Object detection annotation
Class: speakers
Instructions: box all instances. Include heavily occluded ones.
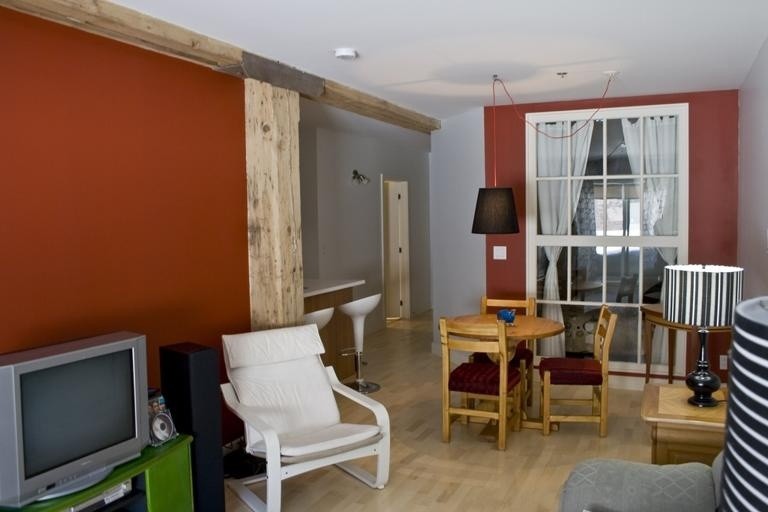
[159,342,225,512]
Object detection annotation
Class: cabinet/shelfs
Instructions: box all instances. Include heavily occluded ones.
[637,381,730,467]
[0,431,197,512]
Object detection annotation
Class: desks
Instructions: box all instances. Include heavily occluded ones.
[637,300,734,384]
[535,273,603,345]
[303,274,375,385]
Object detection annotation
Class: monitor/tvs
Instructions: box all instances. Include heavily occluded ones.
[0,331,150,509]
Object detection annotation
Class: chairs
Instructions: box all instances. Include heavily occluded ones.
[301,306,335,334]
[337,292,385,396]
[216,321,393,512]
[584,274,637,332]
[435,293,627,454]
[554,443,724,511]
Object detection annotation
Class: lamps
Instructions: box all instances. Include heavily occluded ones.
[468,69,523,237]
[710,292,768,511]
[659,263,746,407]
